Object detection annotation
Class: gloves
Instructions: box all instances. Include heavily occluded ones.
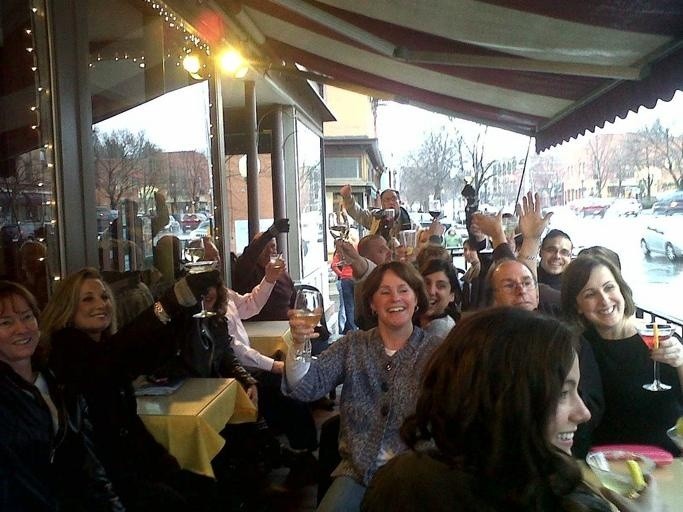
[187,271,218,302]
[269,218,291,236]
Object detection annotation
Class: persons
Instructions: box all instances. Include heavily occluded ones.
[1,184,683,512]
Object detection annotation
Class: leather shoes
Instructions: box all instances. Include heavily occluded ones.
[268,440,308,470]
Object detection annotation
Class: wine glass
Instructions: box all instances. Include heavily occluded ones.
[477,204,503,256]
[429,210,440,223]
[183,238,206,261]
[586,450,657,502]
[288,288,324,364]
[631,317,675,393]
[329,213,354,268]
[181,259,221,320]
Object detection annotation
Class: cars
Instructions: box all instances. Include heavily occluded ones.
[633,216,683,263]
[91,203,216,258]
[1,221,44,247]
[561,194,683,221]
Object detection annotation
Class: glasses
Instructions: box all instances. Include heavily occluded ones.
[544,247,570,257]
[495,277,536,291]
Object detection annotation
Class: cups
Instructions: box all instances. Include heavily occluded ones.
[397,229,417,253]
[382,209,394,224]
[268,253,283,270]
